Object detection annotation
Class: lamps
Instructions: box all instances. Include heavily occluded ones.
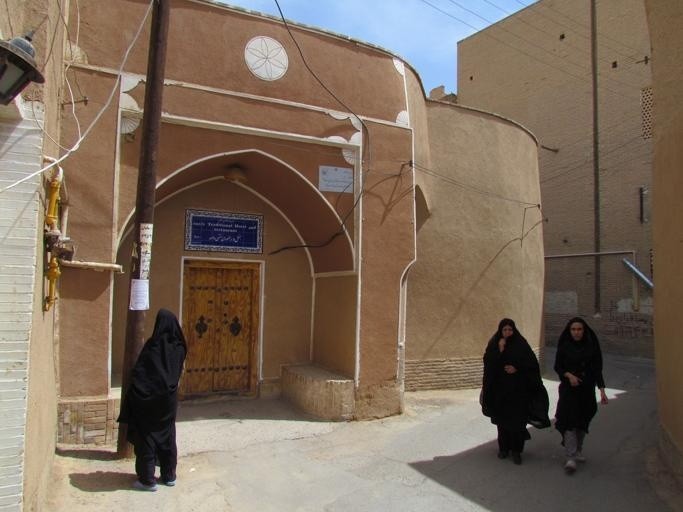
[1,14,47,105]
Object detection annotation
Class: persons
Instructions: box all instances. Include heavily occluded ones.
[115,308,187,492]
[554,317,609,473]
[478,317,551,466]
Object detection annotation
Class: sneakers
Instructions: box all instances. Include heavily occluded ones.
[133,477,175,490]
[564,451,586,475]
[498,449,521,464]
